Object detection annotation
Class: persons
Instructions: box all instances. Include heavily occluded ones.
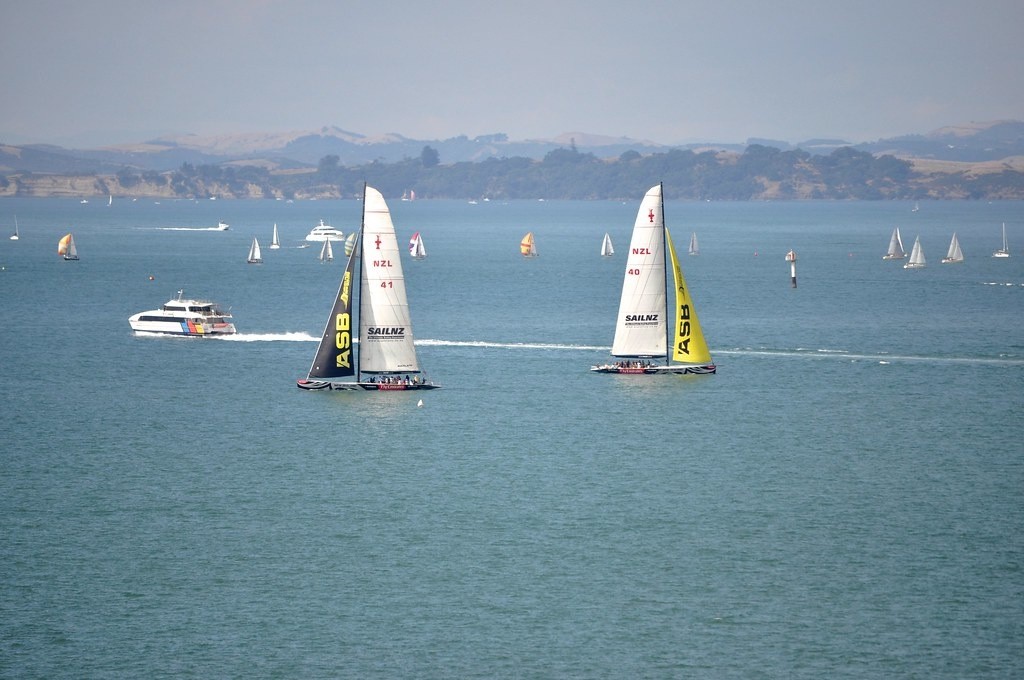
[378,375,401,384]
[370,377,376,382]
[422,378,425,384]
[409,379,417,385]
[615,360,629,368]
[403,375,409,385]
[632,360,650,368]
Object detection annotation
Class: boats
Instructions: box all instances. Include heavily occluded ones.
[304,215,345,242]
[297,242,310,249]
[219,220,230,230]
[128,288,237,336]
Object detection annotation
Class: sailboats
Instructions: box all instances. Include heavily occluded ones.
[408,232,427,258]
[993,222,1010,258]
[106,195,112,207]
[401,187,415,202]
[941,233,964,264]
[521,231,539,257]
[320,236,333,265]
[883,226,907,260]
[270,224,280,250]
[295,181,442,392]
[601,233,615,257]
[247,237,263,263]
[904,235,927,269]
[689,232,701,255]
[58,234,80,260]
[344,232,359,258]
[590,181,717,375]
